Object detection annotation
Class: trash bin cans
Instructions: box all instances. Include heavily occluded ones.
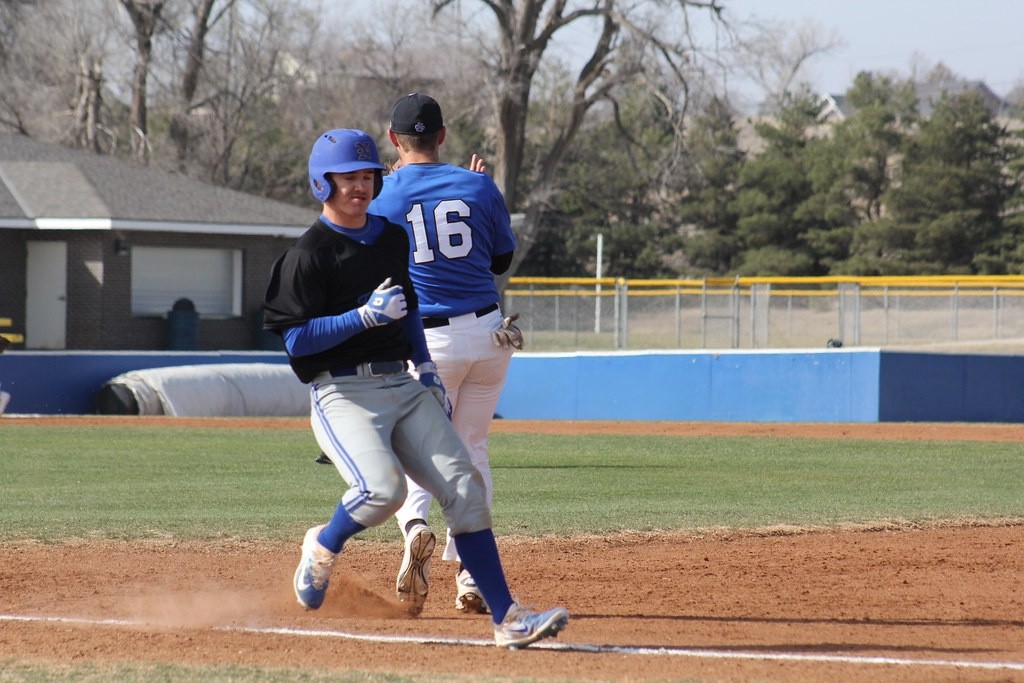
[252,309,283,352]
[166,297,200,351]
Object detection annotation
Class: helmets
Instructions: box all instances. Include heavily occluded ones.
[308,128,386,202]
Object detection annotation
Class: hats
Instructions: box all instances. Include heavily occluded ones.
[389,93,443,134]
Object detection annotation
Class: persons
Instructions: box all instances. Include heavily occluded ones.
[264,129,567,647]
[362,94,518,619]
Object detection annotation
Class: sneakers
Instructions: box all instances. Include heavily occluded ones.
[293,524,343,610]
[455,569,487,612]
[492,598,569,651]
[396,524,436,617]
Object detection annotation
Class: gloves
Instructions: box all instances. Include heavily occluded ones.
[357,277,408,329]
[492,313,524,350]
[416,360,452,422]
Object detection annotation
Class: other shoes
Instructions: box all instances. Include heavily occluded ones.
[316,454,332,464]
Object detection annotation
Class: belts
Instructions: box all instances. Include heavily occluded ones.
[422,303,498,329]
[330,360,408,378]
